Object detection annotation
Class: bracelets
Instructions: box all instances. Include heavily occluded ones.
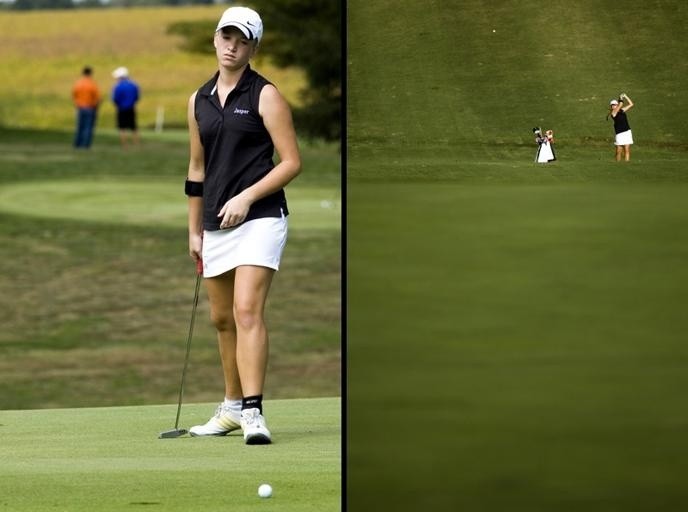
[184,178,203,197]
[619,100,623,102]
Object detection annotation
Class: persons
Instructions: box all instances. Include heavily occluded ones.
[609,92,634,161]
[111,66,140,153]
[183,6,304,444]
[70,65,100,151]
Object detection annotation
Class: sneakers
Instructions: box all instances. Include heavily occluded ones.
[187,404,245,436]
[241,407,271,443]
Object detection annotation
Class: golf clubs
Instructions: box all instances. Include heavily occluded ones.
[606,96,623,123]
[158,257,204,438]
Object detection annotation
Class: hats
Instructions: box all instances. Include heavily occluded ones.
[213,6,263,44]
[112,65,128,78]
[532,126,542,134]
[608,99,618,105]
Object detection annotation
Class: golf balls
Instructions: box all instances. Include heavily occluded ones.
[257,484,272,498]
